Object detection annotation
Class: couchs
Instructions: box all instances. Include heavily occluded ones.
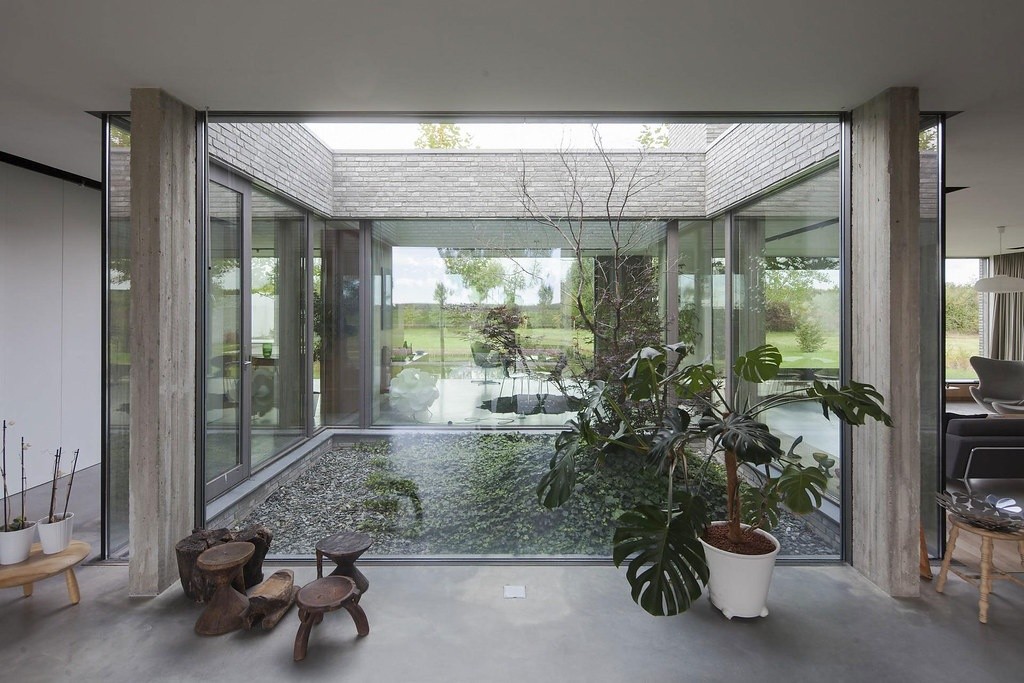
[969,355,1024,413]
[945,412,1024,495]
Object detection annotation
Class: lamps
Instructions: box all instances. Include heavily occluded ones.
[973,226,1024,293]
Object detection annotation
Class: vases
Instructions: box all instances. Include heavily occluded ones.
[0,519,37,566]
[37,512,74,554]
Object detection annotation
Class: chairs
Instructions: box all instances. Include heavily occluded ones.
[471,342,567,405]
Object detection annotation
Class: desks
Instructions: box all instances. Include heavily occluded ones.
[0,540,91,604]
[936,513,1024,623]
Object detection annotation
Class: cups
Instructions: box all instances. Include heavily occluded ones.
[263,343,272,357]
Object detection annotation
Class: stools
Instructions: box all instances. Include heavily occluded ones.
[194,541,256,636]
[293,576,370,660]
[315,532,372,595]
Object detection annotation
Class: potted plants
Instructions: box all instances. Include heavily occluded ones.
[538,340,895,617]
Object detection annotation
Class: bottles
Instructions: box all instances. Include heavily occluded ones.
[392,347,407,361]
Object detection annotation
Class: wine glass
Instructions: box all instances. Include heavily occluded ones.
[408,354,415,361]
